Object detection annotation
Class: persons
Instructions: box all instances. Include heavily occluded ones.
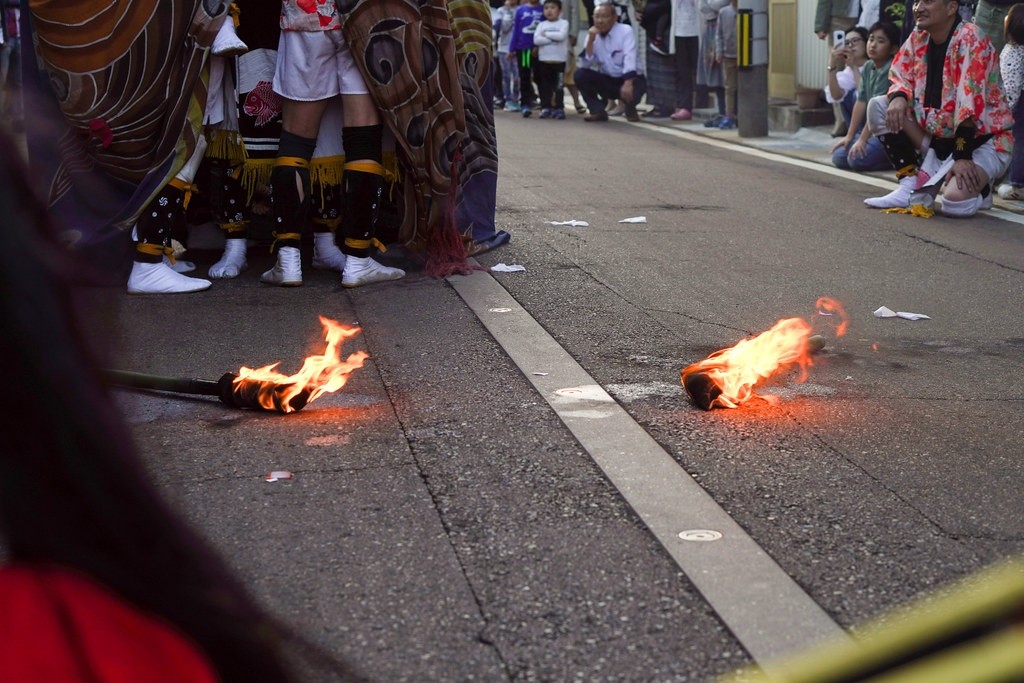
[862,0,1015,217]
[492,0,741,130]
[0,109,370,683]
[812,0,1024,200]
[124,0,405,293]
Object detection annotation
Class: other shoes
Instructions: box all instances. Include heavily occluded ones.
[705,114,736,129]
[670,109,692,120]
[127,254,211,294]
[208,239,248,278]
[342,255,406,287]
[863,175,923,209]
[493,98,565,119]
[313,246,346,269]
[576,105,586,113]
[980,178,995,209]
[583,110,608,122]
[626,107,640,121]
[259,246,305,286]
[997,184,1024,201]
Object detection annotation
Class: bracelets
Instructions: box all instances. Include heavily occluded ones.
[952,125,975,160]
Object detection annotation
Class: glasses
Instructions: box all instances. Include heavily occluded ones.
[845,36,864,47]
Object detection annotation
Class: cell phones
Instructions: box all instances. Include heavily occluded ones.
[833,30,845,49]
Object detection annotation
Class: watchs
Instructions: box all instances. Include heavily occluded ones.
[826,65,836,72]
[887,91,909,101]
